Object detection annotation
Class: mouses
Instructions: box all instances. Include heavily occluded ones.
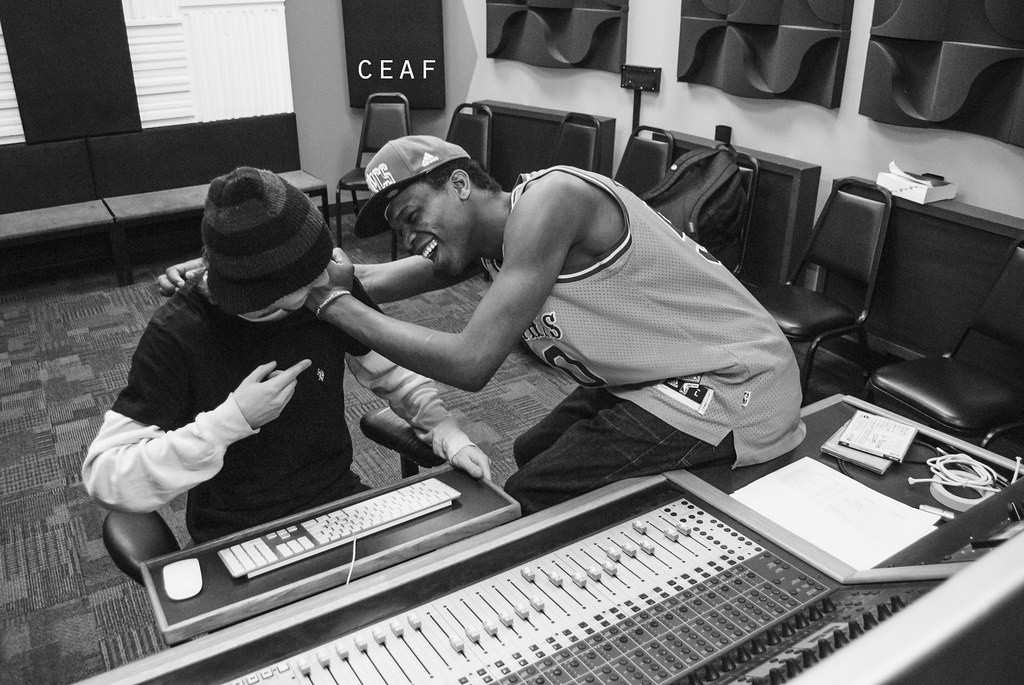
[163,557,203,601]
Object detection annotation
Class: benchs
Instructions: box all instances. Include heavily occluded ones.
[84,113,330,286]
[0,140,126,289]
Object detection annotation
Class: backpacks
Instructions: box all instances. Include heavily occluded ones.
[637,143,748,262]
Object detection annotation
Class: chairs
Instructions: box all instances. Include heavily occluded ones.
[863,237,1024,450]
[548,111,601,171]
[609,125,760,276]
[101,406,448,585]
[752,175,894,407]
[336,91,415,263]
[446,101,494,178]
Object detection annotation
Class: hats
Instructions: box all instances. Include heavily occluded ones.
[353,135,471,237]
[201,166,332,314]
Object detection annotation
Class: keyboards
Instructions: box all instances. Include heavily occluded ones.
[217,477,462,579]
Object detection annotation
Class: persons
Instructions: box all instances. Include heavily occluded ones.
[156,135,806,518]
[80,166,493,545]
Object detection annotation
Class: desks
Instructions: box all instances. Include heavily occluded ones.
[64,395,1024,685]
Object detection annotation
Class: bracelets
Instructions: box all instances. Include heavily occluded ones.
[316,291,351,321]
